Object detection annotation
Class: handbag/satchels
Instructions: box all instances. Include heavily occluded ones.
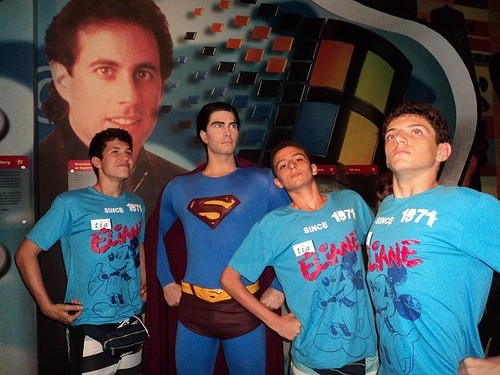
[77,314,148,358]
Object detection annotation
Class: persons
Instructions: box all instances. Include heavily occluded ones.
[36,0,192,375]
[220,140,382,375]
[146,101,295,374]
[14,129,148,375]
[363,101,500,374]
